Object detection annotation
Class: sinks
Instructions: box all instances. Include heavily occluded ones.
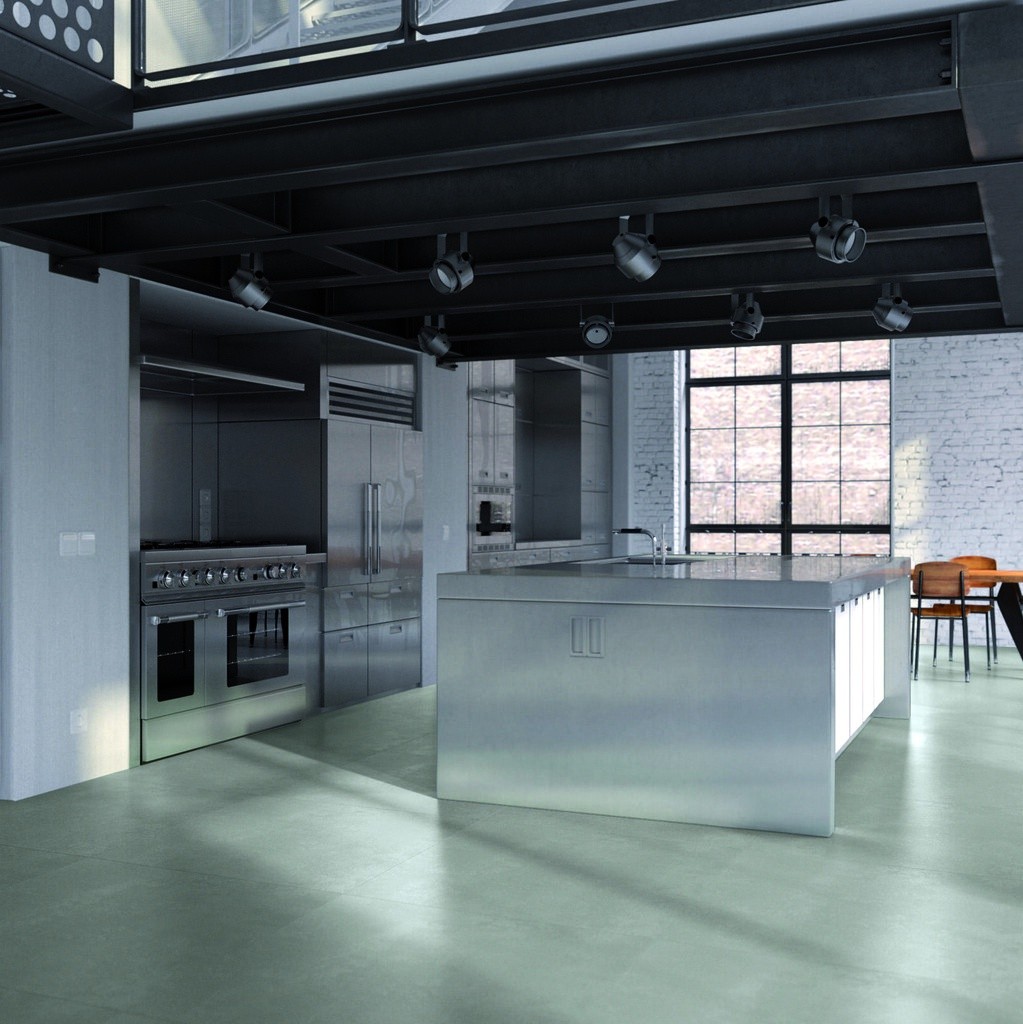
[569,556,708,566]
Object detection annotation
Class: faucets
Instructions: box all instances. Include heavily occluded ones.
[610,528,658,565]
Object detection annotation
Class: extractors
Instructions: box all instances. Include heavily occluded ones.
[138,355,305,397]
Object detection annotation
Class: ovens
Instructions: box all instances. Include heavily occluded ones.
[140,589,306,761]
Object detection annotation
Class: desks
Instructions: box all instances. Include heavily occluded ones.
[909,565,1023,661]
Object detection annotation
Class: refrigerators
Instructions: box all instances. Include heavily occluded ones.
[218,419,423,711]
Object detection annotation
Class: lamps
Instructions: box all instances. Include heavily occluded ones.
[426,230,475,297]
[810,212,866,265]
[871,283,912,331]
[612,212,663,283]
[422,314,454,359]
[729,293,766,341]
[226,248,278,312]
[578,303,615,348]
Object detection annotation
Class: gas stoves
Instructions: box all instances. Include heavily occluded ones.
[140,538,307,589]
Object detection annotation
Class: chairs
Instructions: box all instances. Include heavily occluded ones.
[906,555,998,682]
